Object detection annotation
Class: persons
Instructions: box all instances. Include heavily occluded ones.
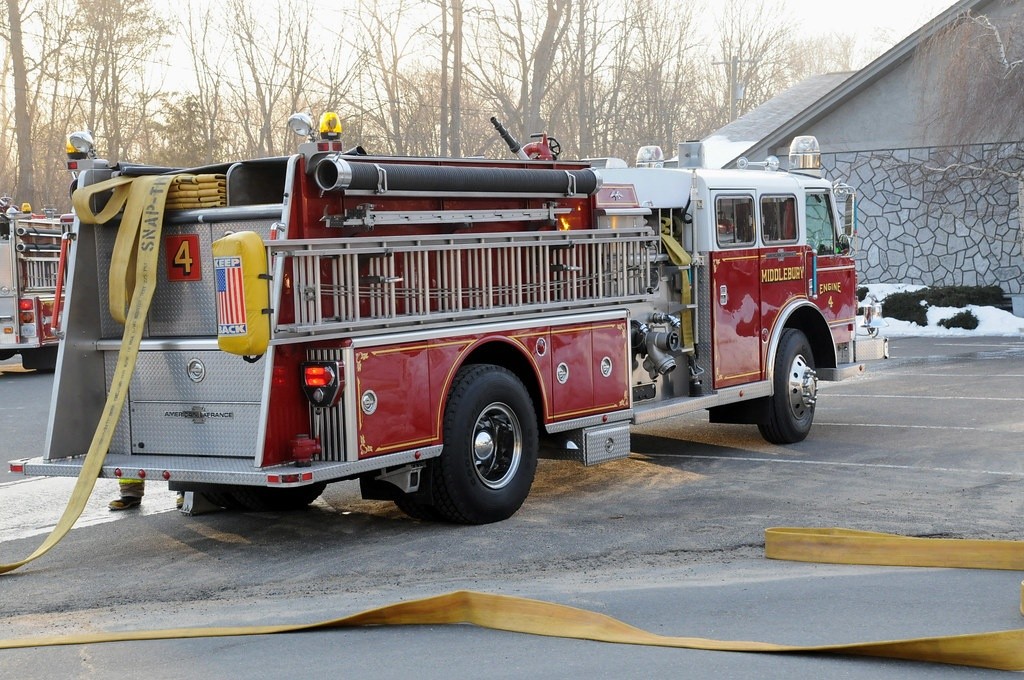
[108,479,184,510]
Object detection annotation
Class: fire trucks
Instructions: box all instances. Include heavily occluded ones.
[0,196,75,373]
[7,113,889,526]
